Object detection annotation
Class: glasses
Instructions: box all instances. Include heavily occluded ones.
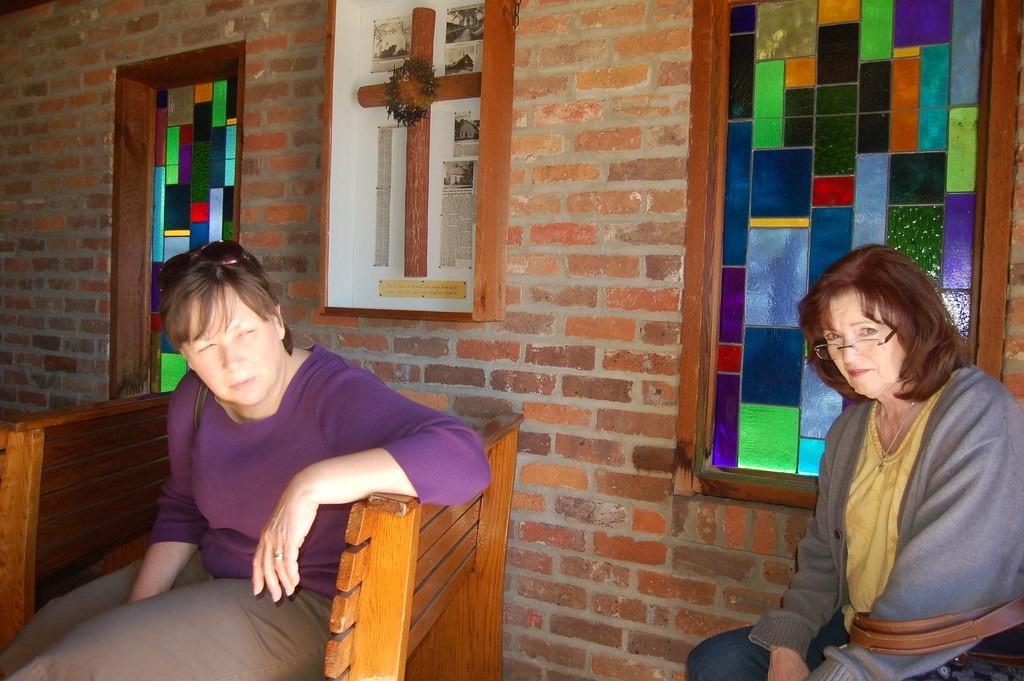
[157,240,268,300]
[814,328,898,360]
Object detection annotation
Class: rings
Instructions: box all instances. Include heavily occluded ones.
[273,552,283,560]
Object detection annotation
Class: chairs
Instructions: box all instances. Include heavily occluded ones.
[0,391,526,681]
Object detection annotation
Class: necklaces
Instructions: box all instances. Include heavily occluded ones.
[879,400,915,472]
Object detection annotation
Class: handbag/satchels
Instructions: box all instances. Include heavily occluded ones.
[849,594,1024,669]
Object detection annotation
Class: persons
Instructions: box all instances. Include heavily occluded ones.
[686,244,1024,681]
[0,241,492,681]
